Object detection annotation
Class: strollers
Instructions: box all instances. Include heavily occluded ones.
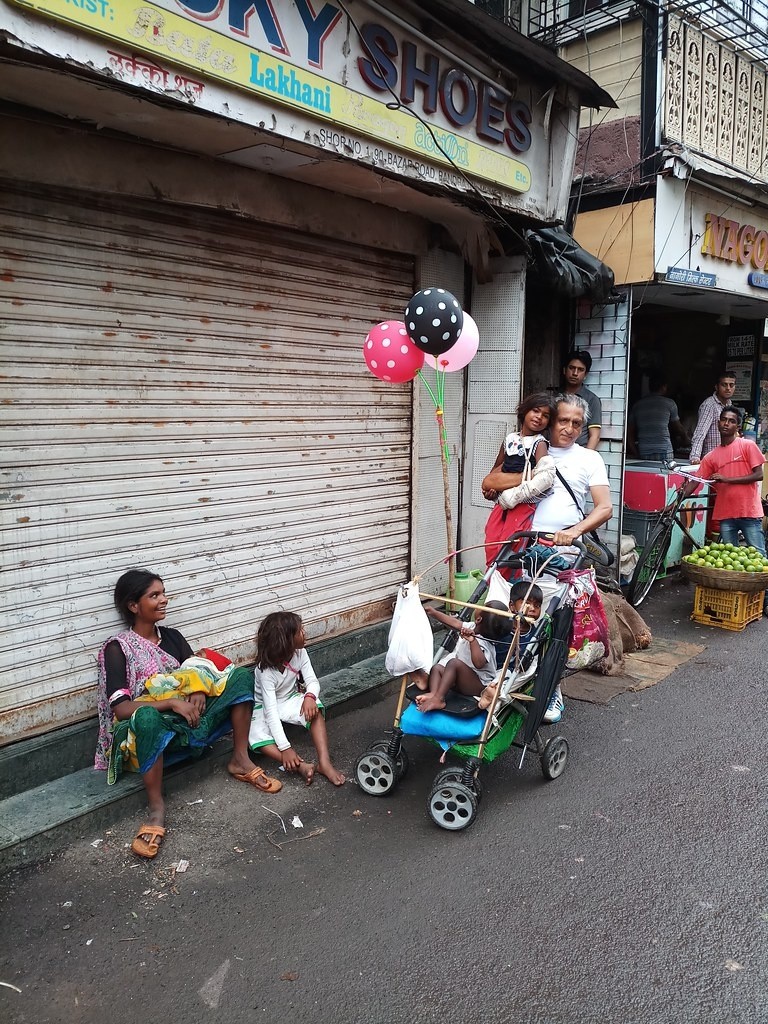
[353,531,588,832]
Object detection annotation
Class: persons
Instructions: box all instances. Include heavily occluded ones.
[247,612,346,785]
[415,581,543,712]
[688,371,735,464]
[539,351,601,450]
[482,391,613,722]
[482,393,556,584]
[627,375,687,460]
[660,406,768,616]
[95,570,282,858]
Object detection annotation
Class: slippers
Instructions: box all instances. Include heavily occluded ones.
[234,767,282,793]
[133,825,165,857]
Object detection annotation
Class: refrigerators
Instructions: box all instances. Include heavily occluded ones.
[624,458,709,567]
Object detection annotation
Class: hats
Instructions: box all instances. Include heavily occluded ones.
[202,648,232,671]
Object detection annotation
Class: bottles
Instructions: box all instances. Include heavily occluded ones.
[742,412,748,428]
[743,412,756,431]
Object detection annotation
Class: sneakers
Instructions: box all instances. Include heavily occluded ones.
[542,690,565,723]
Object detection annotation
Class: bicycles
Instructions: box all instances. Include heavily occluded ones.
[625,459,768,609]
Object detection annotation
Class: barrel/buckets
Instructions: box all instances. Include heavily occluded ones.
[446,569,490,621]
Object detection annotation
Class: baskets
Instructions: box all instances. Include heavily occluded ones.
[680,558,768,591]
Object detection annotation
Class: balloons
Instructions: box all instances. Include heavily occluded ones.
[425,310,479,372]
[363,320,425,384]
[404,287,463,355]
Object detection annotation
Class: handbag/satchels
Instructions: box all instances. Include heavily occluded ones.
[557,568,610,669]
[385,581,434,676]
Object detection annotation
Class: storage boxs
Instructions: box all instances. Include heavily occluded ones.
[692,585,765,632]
[742,431,756,444]
[622,508,670,582]
